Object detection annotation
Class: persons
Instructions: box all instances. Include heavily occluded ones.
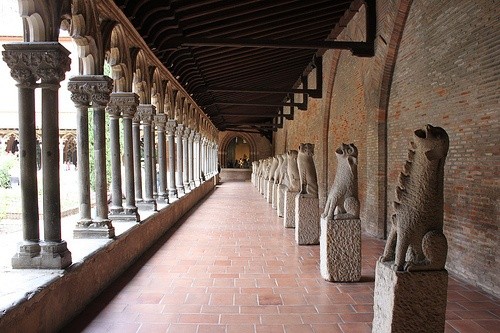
[234,159,238,167]
[238,158,243,167]
